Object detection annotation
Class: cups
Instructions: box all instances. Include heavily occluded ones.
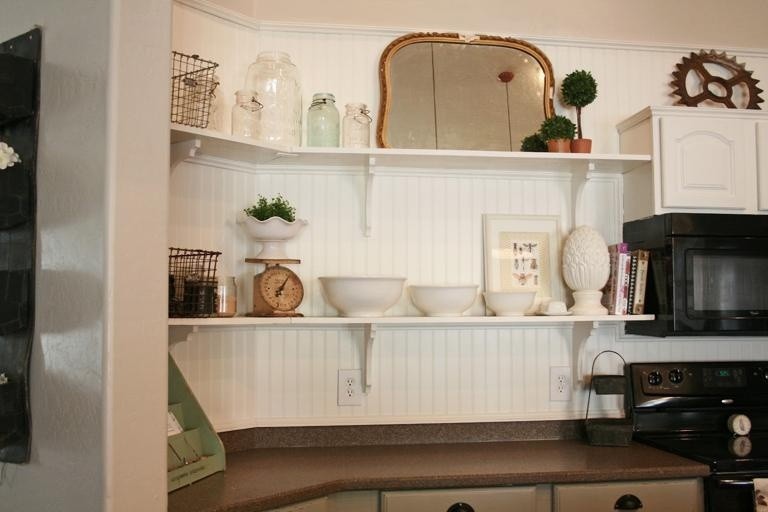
[546,301,567,312]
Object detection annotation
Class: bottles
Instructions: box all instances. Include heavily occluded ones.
[307,92,341,148]
[342,104,373,149]
[232,50,303,148]
[217,277,238,318]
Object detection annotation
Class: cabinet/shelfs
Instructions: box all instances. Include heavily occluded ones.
[170,0,768,512]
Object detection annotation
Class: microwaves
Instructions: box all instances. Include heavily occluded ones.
[624,213,768,339]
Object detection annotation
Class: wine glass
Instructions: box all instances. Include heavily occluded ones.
[236,215,307,259]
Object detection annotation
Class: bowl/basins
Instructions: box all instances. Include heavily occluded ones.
[407,284,480,317]
[482,291,535,317]
[318,276,408,316]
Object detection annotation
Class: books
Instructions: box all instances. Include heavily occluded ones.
[601,242,650,315]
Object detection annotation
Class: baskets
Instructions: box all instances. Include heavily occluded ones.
[171,51,219,128]
[169,247,222,319]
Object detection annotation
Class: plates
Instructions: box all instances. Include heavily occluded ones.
[537,311,575,316]
